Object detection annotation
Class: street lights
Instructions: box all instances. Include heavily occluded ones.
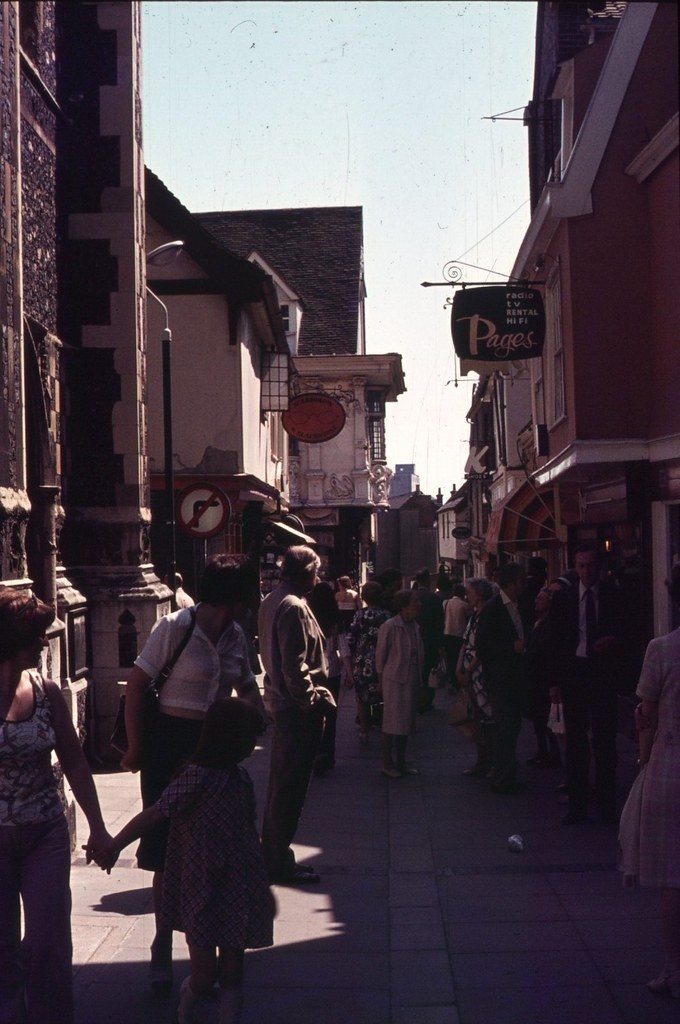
[146,240,185,611]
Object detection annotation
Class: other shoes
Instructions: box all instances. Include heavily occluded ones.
[491,768,517,783]
[602,806,617,823]
[416,702,434,715]
[528,750,560,769]
[358,724,367,738]
[463,758,488,773]
[265,862,321,885]
[380,762,400,777]
[256,720,271,734]
[560,810,589,824]
[312,758,329,777]
[327,759,336,768]
[149,939,173,990]
[399,763,420,776]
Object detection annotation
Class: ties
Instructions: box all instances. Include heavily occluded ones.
[584,589,598,665]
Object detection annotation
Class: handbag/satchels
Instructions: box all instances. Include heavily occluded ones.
[448,687,474,726]
[427,656,445,687]
[108,682,160,755]
[544,702,567,755]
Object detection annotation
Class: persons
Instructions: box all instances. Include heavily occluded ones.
[84,697,276,1024]
[414,569,444,713]
[308,582,353,770]
[335,576,362,633]
[477,538,638,823]
[257,545,337,885]
[619,561,680,999]
[120,554,275,989]
[347,582,395,740]
[457,578,493,774]
[442,579,474,692]
[376,590,424,778]
[373,570,401,612]
[0,588,120,1024]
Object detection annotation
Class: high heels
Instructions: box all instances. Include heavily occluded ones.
[646,969,680,998]
